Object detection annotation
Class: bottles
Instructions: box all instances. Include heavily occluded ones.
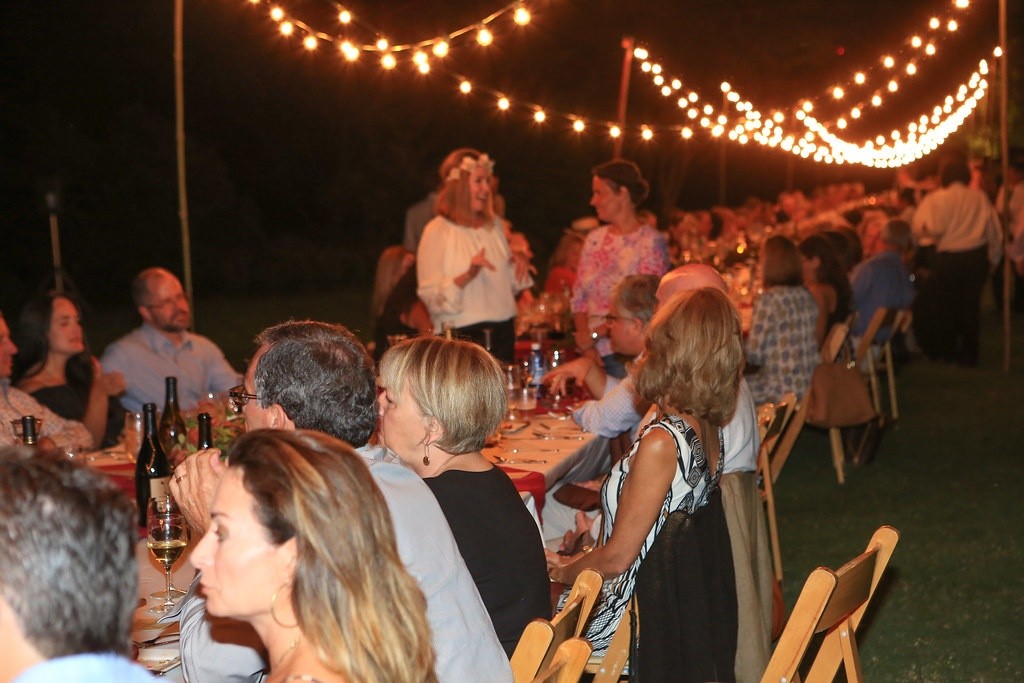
[160,376,187,455]
[197,413,214,453]
[22,415,37,447]
[136,403,170,528]
[483,277,577,408]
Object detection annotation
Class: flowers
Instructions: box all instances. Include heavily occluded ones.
[165,399,243,466]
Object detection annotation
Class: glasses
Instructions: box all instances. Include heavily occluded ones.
[229,385,256,407]
[606,313,633,324]
[145,292,185,309]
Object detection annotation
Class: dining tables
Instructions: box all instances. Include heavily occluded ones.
[79,264,762,683]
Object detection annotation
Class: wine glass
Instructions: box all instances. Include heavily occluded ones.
[145,513,189,616]
[147,497,188,600]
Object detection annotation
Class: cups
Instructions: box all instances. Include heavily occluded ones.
[52,422,80,459]
[123,412,144,460]
[9,418,43,447]
[198,392,228,426]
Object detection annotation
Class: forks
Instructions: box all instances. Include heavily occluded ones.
[132,639,182,648]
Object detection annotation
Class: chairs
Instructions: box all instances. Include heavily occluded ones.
[759,525,901,683]
[582,593,639,683]
[509,567,605,683]
[755,392,799,581]
[854,306,903,424]
[529,636,594,683]
[769,321,850,485]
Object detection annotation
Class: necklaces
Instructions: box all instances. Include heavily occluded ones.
[272,635,302,671]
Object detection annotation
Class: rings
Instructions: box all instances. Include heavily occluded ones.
[176,474,188,484]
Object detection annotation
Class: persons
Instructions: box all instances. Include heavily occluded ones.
[0,147,1023,683]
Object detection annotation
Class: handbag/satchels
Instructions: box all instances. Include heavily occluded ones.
[806,323,878,426]
